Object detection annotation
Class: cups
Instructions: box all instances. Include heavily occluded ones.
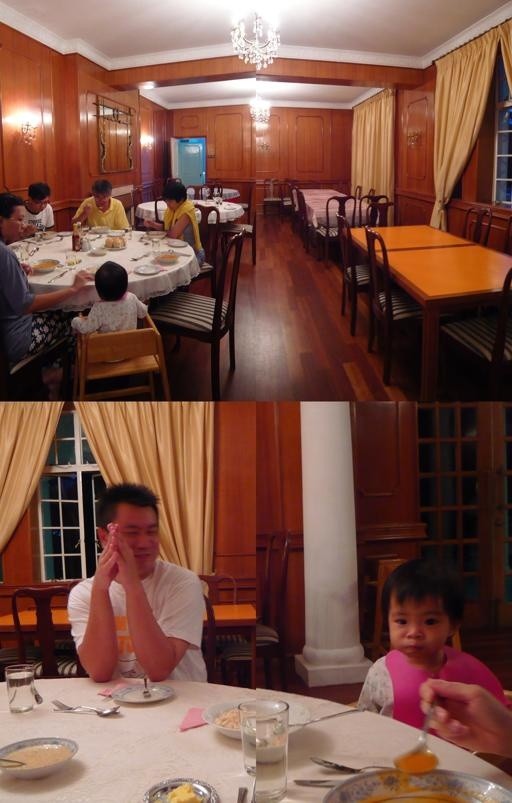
[253,698,290,803]
[4,663,36,714]
[237,701,256,775]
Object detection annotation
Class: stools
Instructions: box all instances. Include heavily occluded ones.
[360,556,463,662]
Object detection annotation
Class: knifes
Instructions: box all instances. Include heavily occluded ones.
[30,685,43,704]
[293,778,344,788]
[47,269,69,284]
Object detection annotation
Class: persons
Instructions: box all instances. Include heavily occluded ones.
[71,179,130,233]
[142,181,206,275]
[70,260,148,365]
[1,194,94,400]
[67,483,208,683]
[11,182,56,243]
[354,557,511,769]
[419,679,512,758]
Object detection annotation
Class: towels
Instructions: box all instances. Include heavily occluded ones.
[178,707,208,731]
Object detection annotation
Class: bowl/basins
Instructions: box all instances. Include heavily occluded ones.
[1,737,79,779]
[319,769,512,802]
[282,700,310,735]
[200,699,242,740]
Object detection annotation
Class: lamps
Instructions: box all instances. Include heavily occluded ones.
[21,118,40,145]
[232,11,282,69]
[248,95,271,131]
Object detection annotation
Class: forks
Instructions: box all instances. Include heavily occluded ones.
[142,676,152,697]
[52,698,103,713]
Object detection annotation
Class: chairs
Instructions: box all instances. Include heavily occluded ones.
[1,528,294,696]
[1,174,512,401]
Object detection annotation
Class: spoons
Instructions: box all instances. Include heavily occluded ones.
[312,756,394,772]
[394,699,437,775]
[52,705,120,717]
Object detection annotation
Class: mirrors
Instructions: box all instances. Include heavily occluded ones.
[93,92,137,174]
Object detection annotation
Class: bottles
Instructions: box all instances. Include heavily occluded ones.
[72,222,82,250]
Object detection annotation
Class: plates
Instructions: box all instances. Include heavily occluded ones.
[143,778,221,803]
[23,229,189,275]
[109,684,175,704]
[224,205,236,209]
